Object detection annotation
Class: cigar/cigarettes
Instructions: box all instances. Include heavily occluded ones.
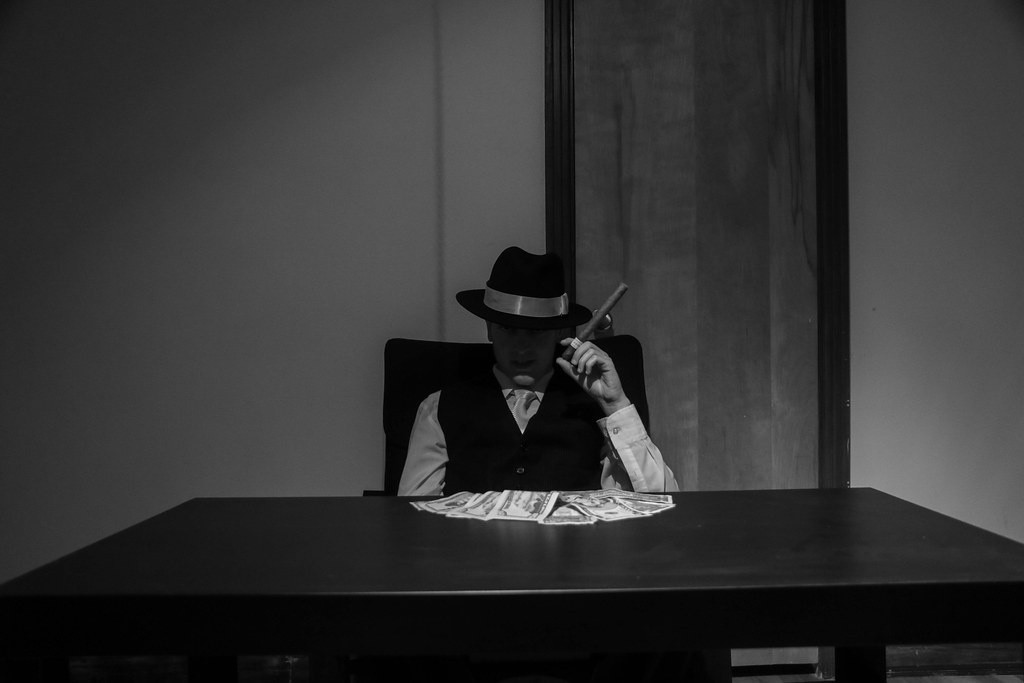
[563,282,629,360]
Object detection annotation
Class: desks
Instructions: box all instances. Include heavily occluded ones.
[0,484,1024,683]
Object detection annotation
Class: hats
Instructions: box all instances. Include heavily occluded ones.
[455,246,593,330]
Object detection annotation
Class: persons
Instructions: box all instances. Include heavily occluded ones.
[396,246,681,496]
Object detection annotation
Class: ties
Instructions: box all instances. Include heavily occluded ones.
[511,388,537,434]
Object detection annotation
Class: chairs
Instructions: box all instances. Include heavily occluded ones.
[364,333,652,498]
